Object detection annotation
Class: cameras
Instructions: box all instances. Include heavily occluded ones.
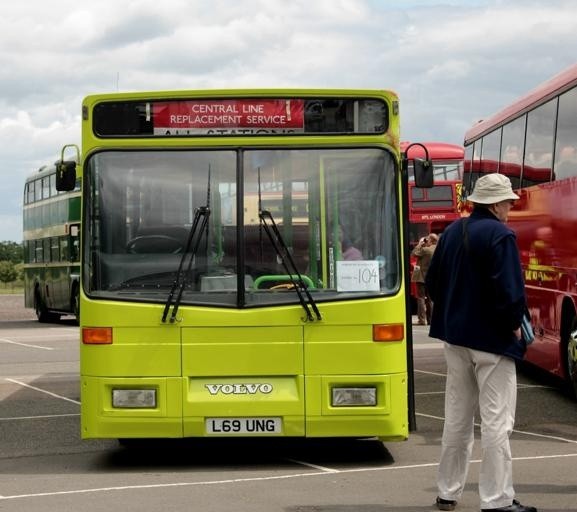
[424,238,428,243]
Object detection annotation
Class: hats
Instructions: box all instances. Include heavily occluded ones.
[465,173,521,205]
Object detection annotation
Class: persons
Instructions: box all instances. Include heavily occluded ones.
[421,171,538,511]
[304,218,363,280]
[412,232,440,325]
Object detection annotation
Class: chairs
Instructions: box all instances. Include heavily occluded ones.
[129,224,310,279]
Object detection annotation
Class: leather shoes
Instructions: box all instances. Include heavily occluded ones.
[434,495,459,511]
[480,495,539,512]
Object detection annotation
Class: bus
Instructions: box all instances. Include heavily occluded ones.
[461,64,577,402]
[398,141,464,316]
[462,159,557,197]
[231,191,309,227]
[55,88,434,448]
[22,154,83,324]
